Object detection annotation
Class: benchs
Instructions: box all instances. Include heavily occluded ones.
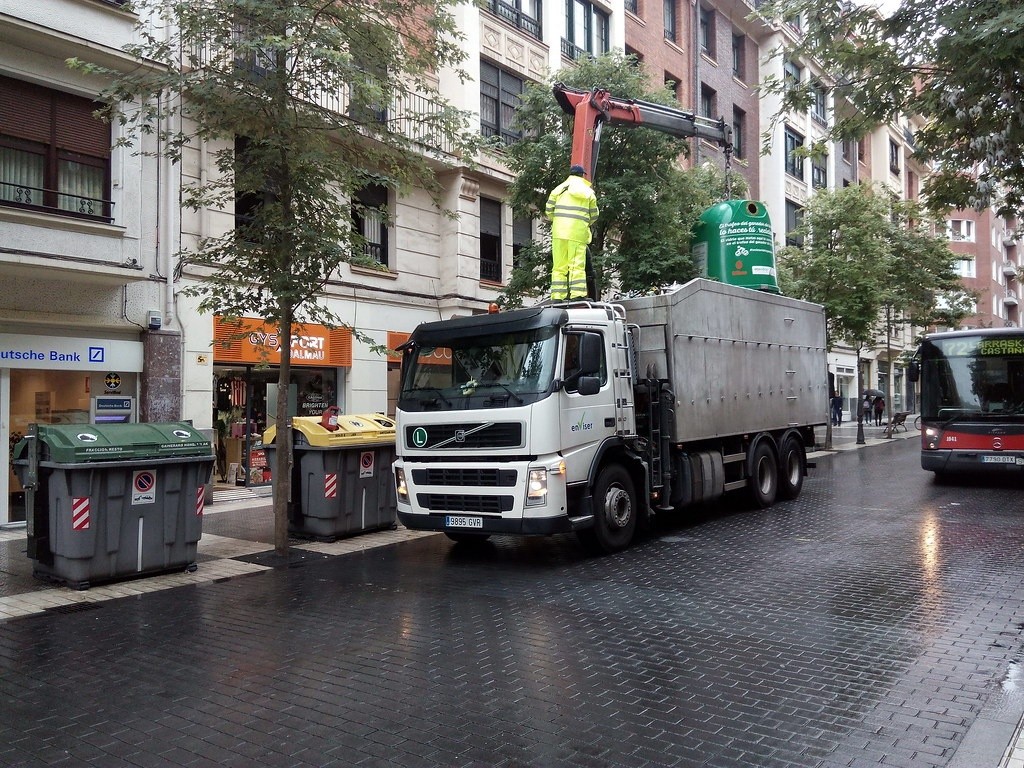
[882,412,909,433]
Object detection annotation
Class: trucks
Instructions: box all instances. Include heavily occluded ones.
[385,91,835,554]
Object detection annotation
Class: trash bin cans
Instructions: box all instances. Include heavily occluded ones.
[11,420,216,591]
[260,405,398,544]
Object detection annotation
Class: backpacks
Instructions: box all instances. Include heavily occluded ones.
[863,401,869,408]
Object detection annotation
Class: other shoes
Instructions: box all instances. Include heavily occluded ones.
[865,423,882,426]
[551,296,589,309]
[834,422,841,426]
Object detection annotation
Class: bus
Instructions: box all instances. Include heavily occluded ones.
[909,325,1024,473]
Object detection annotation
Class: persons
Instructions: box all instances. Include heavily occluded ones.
[831,391,842,426]
[873,397,885,426]
[545,166,599,298]
[864,396,873,425]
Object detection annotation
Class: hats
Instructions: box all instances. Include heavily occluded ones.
[570,165,589,176]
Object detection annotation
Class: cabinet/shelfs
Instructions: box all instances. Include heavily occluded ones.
[226,436,262,480]
[231,422,257,438]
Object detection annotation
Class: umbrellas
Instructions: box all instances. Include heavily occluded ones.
[862,388,886,398]
